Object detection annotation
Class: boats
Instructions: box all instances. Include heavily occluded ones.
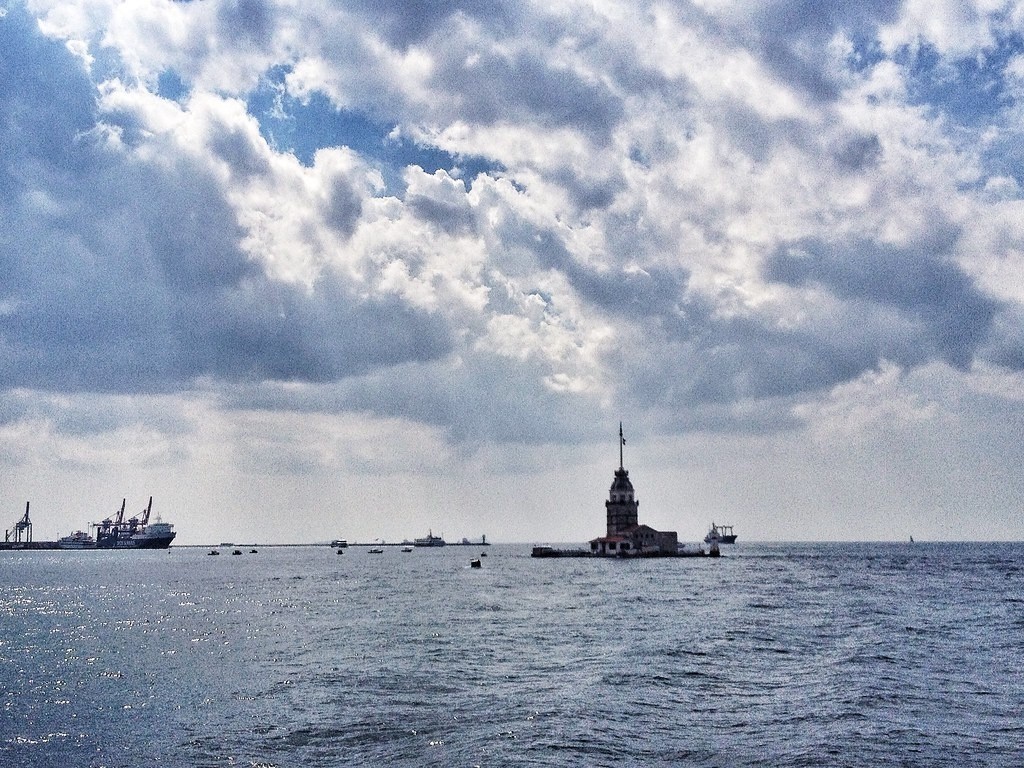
[330,540,348,549]
[368,547,383,553]
[414,529,446,547]
[401,547,412,553]
[703,520,738,544]
[55,494,177,550]
